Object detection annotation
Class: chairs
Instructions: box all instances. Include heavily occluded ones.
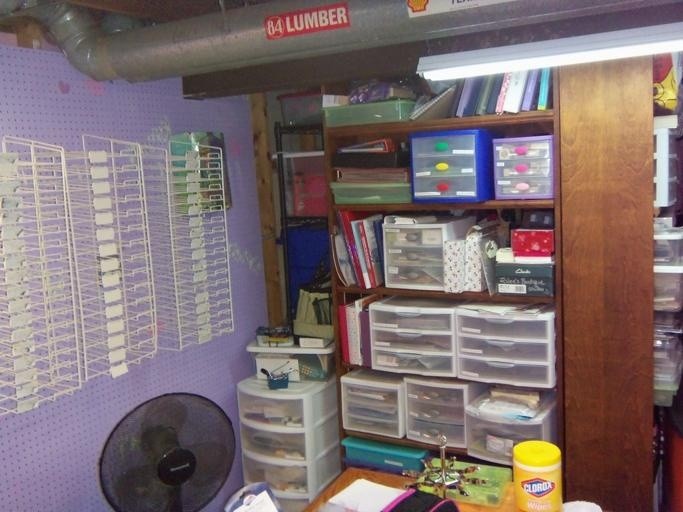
[222,480,283,512]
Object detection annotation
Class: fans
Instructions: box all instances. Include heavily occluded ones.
[97,392,235,512]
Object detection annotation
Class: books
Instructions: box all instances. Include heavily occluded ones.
[327,478,405,512]
[330,209,385,292]
[447,67,551,118]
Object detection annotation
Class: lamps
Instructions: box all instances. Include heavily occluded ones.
[415,22,683,81]
